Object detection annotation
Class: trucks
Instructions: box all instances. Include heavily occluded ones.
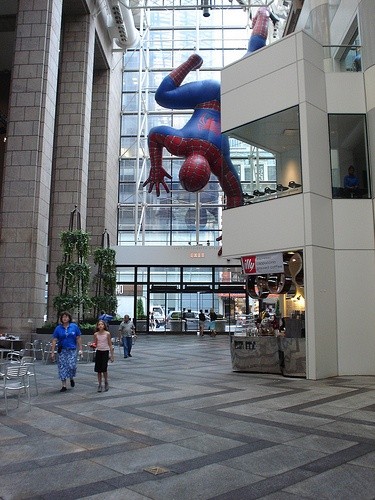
[148,306,168,328]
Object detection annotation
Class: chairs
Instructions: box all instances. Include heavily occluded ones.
[0,339,90,417]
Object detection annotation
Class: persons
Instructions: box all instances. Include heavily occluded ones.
[199,310,206,336]
[98,310,113,331]
[142,7,269,257]
[207,309,217,337]
[264,311,270,318]
[51,311,83,392]
[255,315,262,328]
[118,315,135,358]
[89,320,113,392]
[343,165,359,189]
[182,308,186,319]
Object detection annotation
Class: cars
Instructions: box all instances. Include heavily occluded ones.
[165,311,211,330]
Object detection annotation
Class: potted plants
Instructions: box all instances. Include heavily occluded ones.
[135,297,147,333]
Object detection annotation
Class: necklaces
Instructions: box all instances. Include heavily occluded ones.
[151,313,156,332]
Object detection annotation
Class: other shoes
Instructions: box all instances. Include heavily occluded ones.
[105,382,109,391]
[59,387,67,392]
[124,355,127,358]
[128,354,132,357]
[97,386,102,392]
[70,379,75,387]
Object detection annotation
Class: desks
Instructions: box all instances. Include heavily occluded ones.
[0,348,14,364]
[0,336,26,362]
